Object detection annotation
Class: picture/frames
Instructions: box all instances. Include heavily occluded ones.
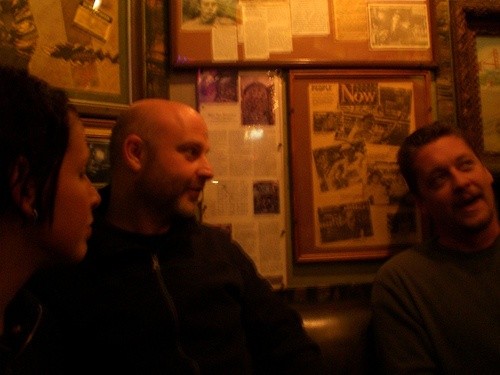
[449,0,499,172]
[167,0,441,68]
[0,0,138,119]
[285,69,433,265]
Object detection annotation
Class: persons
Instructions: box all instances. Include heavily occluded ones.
[183,0,239,29]
[5,98,334,375]
[384,14,406,43]
[0,66,102,375]
[370,119,500,375]
[336,204,368,239]
